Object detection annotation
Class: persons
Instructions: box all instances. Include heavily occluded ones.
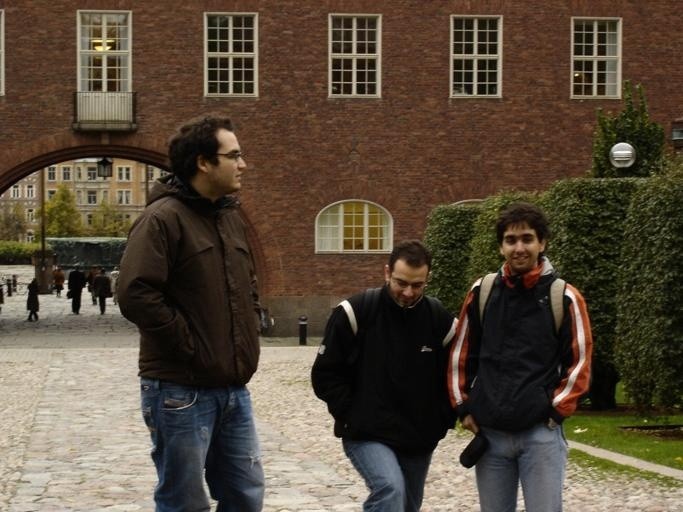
[444,202,596,512]
[87,266,98,306]
[110,110,267,511]
[110,266,120,306]
[25,277,39,322]
[52,266,65,296]
[308,238,461,511]
[91,268,114,315]
[67,262,86,315]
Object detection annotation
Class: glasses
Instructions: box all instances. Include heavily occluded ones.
[217,152,242,158]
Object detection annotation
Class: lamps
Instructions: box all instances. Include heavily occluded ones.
[97,155,114,180]
